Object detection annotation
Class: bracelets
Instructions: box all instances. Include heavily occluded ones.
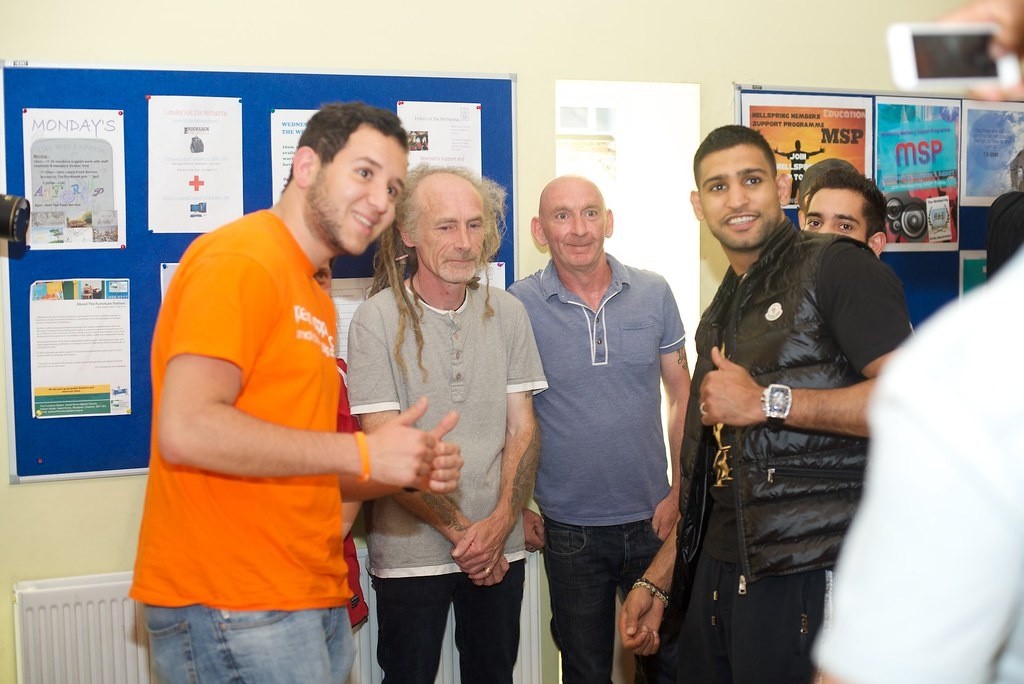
[356,430,371,483]
[633,578,669,608]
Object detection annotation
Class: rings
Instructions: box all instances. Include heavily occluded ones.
[526,545,533,550]
[700,402,707,415]
[483,567,491,574]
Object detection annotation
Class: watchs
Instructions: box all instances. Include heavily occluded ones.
[761,382,792,433]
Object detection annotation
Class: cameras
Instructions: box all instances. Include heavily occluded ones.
[0,194,29,242]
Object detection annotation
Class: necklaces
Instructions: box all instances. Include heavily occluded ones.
[410,271,467,313]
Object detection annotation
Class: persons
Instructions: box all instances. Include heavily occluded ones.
[346,162,550,684]
[813,0,1023,684]
[130,103,467,684]
[790,154,920,333]
[619,124,914,684]
[505,174,694,684]
